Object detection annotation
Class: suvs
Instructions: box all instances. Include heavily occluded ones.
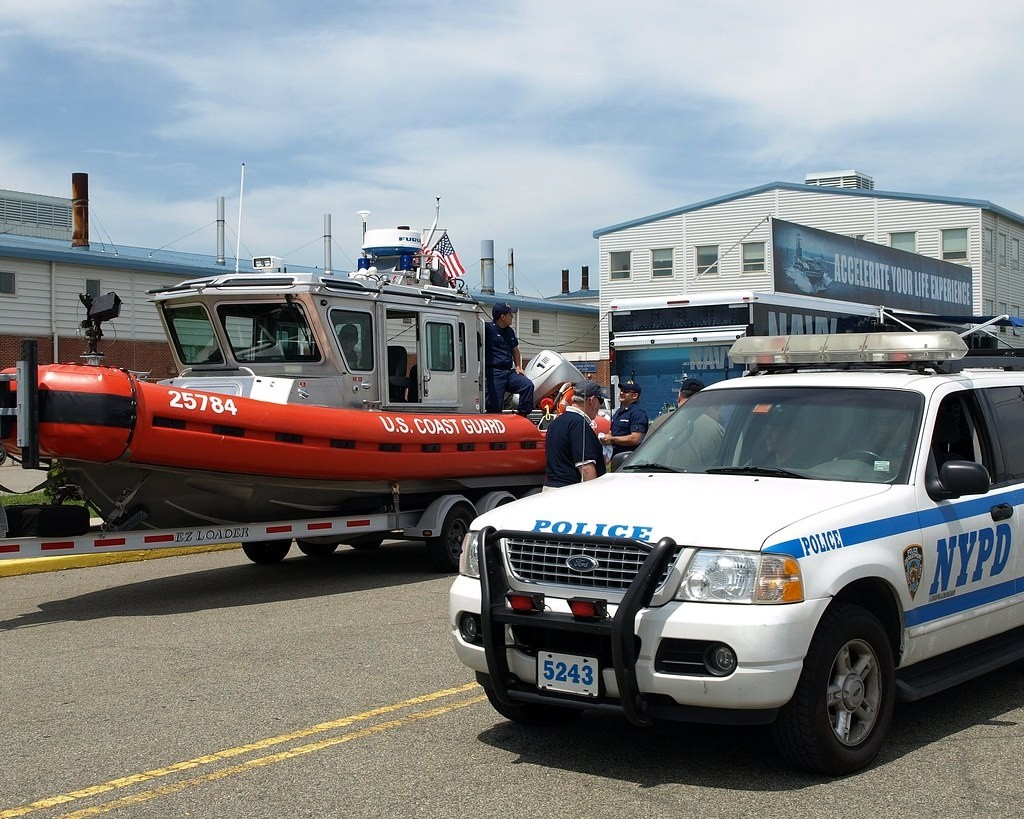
[450,331,1024,781]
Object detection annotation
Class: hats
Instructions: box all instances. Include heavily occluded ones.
[576,380,609,398]
[618,380,640,395]
[681,379,705,392]
[492,302,518,315]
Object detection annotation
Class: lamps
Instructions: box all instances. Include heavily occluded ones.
[251,256,283,273]
[417,267,430,286]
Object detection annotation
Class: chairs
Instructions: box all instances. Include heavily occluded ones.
[384,347,411,402]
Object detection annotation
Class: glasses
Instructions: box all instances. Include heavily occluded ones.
[621,389,636,394]
[594,396,604,404]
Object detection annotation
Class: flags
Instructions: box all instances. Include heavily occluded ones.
[415,232,465,287]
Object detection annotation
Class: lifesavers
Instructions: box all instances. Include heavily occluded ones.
[559,389,573,413]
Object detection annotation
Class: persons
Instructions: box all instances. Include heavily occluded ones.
[601,381,648,462]
[339,324,359,369]
[485,302,534,418]
[704,402,725,428]
[642,379,726,474]
[542,380,610,491]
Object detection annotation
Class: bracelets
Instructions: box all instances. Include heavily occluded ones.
[611,437,616,447]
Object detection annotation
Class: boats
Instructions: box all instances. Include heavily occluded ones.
[0,226,614,526]
